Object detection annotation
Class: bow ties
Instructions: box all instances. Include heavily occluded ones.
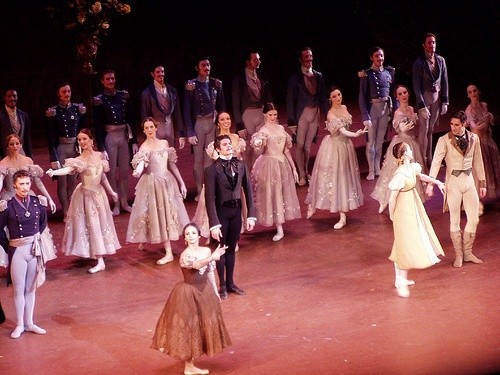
[221,156,239,179]
[455,134,468,148]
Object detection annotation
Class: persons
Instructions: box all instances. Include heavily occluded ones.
[168,223,229,375]
[306,86,369,230]
[91,68,139,216]
[182,55,227,201]
[426,110,487,268]
[140,64,186,158]
[46,128,119,273]
[388,141,446,298]
[0,170,48,338]
[203,134,257,300]
[0,134,57,270]
[249,101,300,242]
[197,111,246,253]
[0,87,35,161]
[131,116,187,265]
[357,47,395,180]
[287,47,329,185]
[377,84,425,214]
[412,32,450,169]
[44,82,87,222]
[231,48,271,169]
[464,82,500,212]
